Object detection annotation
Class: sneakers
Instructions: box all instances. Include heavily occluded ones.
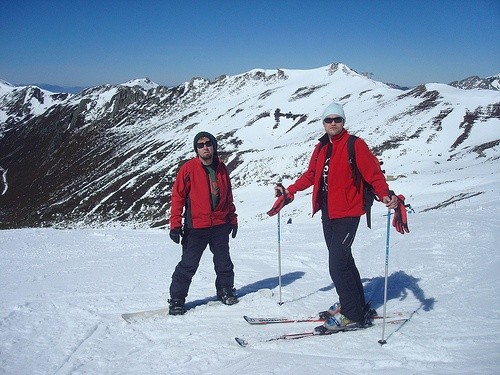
[217,287,237,304]
[324,311,367,329]
[167,298,185,315]
[318,301,370,318]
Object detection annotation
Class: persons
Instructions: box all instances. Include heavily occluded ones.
[168,132,239,314]
[275,101,399,331]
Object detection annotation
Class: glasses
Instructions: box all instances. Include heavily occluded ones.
[196,141,211,148]
[325,117,342,123]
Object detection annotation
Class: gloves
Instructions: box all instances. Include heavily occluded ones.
[170,226,184,243]
[266,195,294,217]
[228,224,238,238]
[392,194,411,234]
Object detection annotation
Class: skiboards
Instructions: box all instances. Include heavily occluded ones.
[235,310,415,349]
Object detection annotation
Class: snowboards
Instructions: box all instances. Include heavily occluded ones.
[121,287,275,325]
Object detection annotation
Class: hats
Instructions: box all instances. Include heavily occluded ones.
[322,103,346,124]
